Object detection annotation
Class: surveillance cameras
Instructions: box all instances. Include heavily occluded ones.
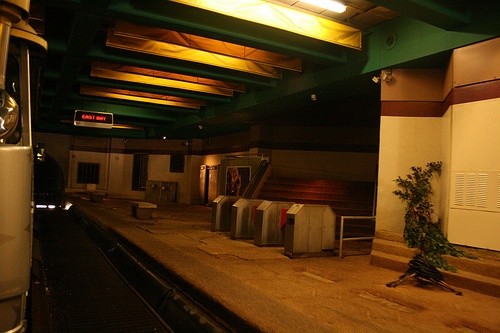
[372,76,381,84]
[310,94,317,101]
[383,73,393,82]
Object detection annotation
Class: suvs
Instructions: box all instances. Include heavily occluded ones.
[33,150,65,215]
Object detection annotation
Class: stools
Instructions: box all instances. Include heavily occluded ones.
[130,201,157,219]
[90,192,106,202]
